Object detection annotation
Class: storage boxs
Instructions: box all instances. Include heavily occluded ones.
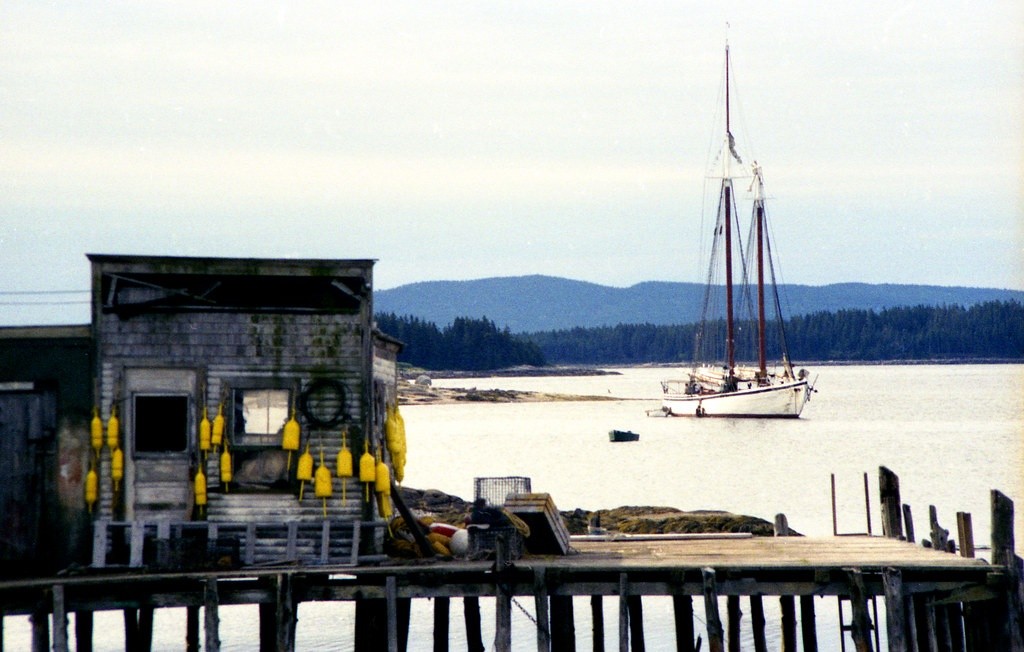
[505,492,571,557]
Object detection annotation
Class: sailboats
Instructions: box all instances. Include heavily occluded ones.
[657,18,820,420]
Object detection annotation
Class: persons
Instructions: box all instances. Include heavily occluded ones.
[685,383,703,395]
[722,368,750,392]
